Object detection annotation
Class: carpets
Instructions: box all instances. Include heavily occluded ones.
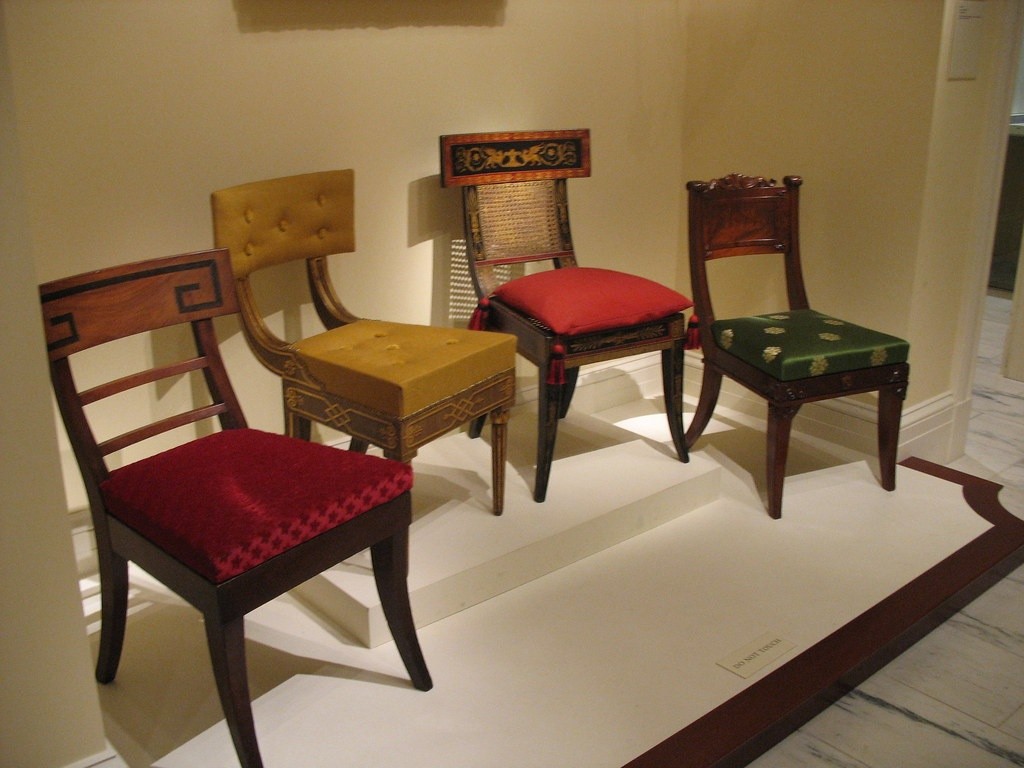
[77,388,1024,768]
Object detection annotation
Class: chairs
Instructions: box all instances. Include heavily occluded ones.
[437,125,692,501]
[683,171,913,520]
[29,247,414,768]
[206,169,521,517]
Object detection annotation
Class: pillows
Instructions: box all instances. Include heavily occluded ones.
[493,264,698,338]
